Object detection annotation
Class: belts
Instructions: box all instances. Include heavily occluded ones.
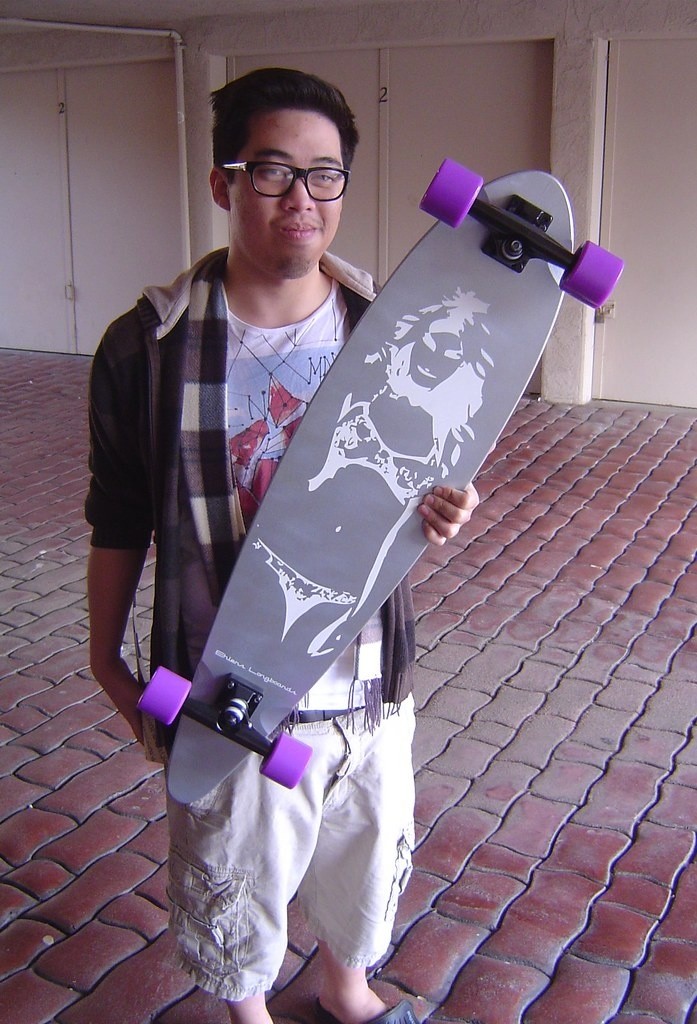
[290,706,365,725]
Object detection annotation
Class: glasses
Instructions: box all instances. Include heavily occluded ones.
[222,160,351,201]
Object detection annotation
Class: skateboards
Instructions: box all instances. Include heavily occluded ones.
[138,159,626,811]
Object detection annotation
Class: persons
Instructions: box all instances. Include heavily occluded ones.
[80,64,482,1023]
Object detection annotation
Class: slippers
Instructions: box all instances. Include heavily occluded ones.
[314,995,420,1024]
